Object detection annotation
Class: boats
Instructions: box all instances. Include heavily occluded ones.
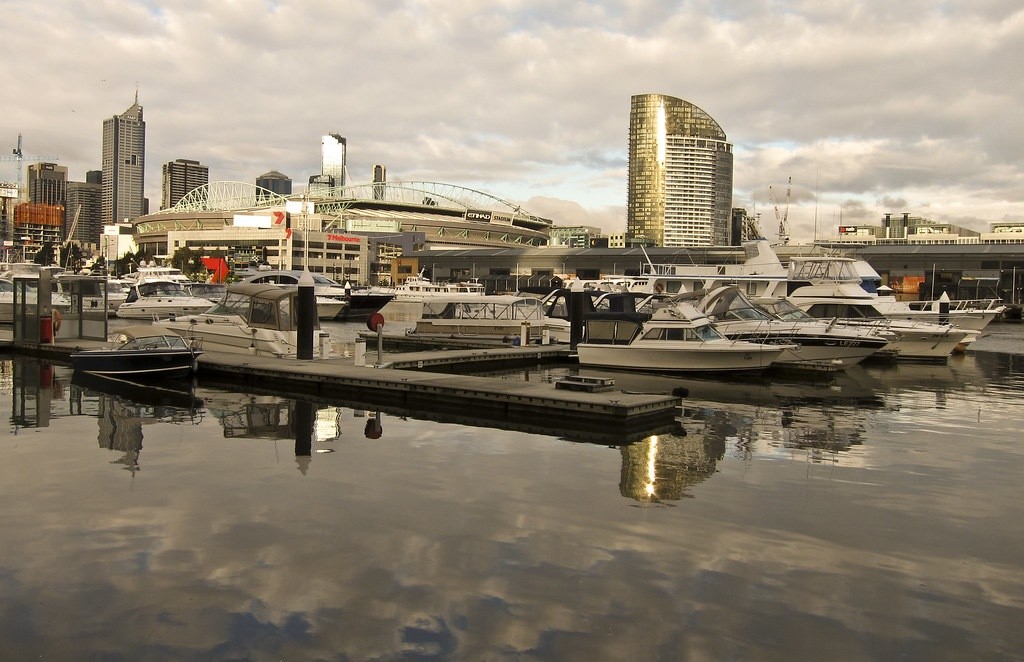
[106,267,191,309]
[390,268,485,303]
[576,302,797,372]
[69,325,204,374]
[218,296,346,318]
[456,286,670,344]
[116,280,216,319]
[314,293,395,321]
[152,281,327,358]
[663,256,1007,372]
[71,368,204,425]
[405,296,547,339]
[0,262,70,321]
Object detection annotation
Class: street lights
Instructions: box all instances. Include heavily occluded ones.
[345,272,350,284]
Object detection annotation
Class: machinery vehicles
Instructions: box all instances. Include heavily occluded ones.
[66,204,81,245]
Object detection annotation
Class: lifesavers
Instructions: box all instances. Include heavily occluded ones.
[655,284,664,292]
[50,308,62,331]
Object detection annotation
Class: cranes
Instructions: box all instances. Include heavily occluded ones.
[770,186,785,236]
[784,177,791,235]
[0,136,59,190]
